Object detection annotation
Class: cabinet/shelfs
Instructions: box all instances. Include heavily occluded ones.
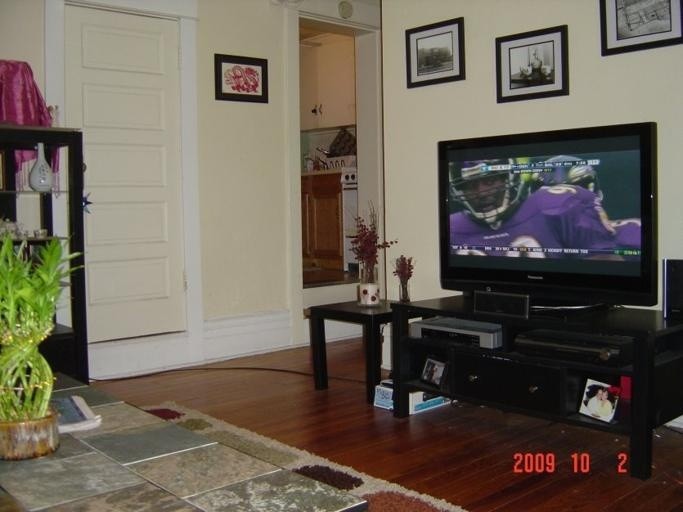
[0,123,85,389]
[302,169,344,272]
[299,34,356,132]
[389,292,683,480]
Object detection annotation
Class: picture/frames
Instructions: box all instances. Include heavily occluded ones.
[494,24,569,104]
[213,53,271,105]
[417,353,450,390]
[576,376,619,423]
[405,15,466,90]
[598,1,683,57]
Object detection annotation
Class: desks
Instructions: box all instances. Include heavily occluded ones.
[0,394,367,512]
[302,297,435,400]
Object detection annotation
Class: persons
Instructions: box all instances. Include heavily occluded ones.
[587,388,613,418]
[450,150,642,260]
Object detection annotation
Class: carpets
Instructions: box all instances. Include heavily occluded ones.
[136,398,472,512]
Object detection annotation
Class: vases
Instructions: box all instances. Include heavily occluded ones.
[361,266,378,284]
[400,280,409,302]
[26,142,53,193]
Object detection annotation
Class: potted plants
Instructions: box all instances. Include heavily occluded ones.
[0,231,63,462]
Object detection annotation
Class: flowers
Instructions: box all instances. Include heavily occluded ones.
[391,253,415,300]
[346,204,397,281]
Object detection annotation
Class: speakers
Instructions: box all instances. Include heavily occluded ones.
[662,259,683,319]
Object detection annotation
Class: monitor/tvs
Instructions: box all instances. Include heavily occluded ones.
[437,121,657,309]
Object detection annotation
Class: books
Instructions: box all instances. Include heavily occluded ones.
[48,394,102,434]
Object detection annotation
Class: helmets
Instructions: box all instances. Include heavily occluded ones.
[530,153,603,201]
[448,156,525,230]
